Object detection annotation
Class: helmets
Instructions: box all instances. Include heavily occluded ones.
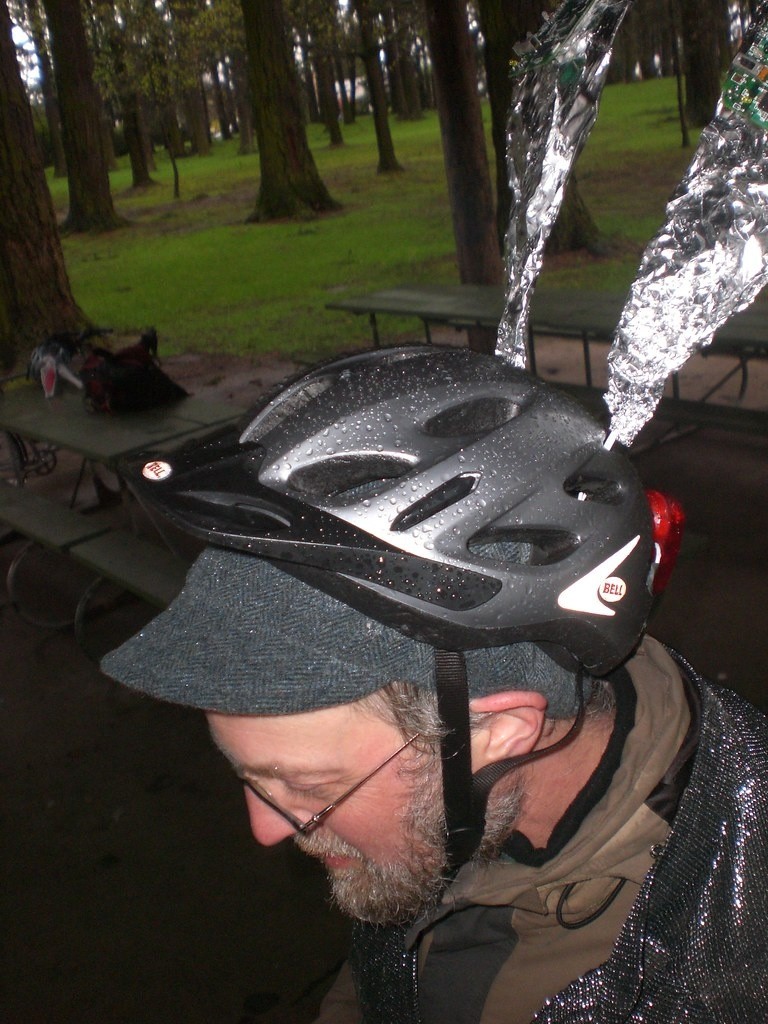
[103,344,656,862]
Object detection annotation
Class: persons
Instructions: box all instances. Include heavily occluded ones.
[98,341,767,1024]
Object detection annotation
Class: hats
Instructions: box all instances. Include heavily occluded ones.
[99,477,597,718]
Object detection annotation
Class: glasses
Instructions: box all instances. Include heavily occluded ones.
[233,734,424,836]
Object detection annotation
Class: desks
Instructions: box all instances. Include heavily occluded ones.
[0,382,251,506]
[328,270,768,402]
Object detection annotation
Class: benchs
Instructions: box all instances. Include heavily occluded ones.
[0,476,197,628]
